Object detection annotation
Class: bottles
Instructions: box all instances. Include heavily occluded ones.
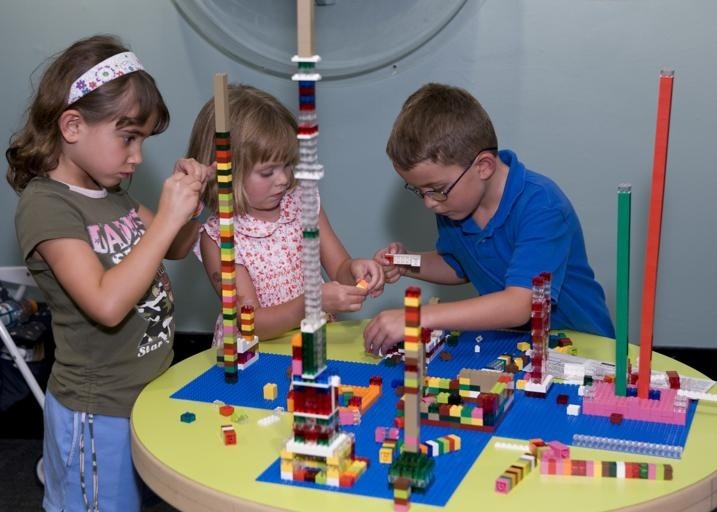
[19,297,38,324]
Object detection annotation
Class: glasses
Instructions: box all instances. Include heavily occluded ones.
[403,146,500,204]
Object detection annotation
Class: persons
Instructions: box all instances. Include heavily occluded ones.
[362,81,617,353]
[185,82,387,348]
[4,32,219,510]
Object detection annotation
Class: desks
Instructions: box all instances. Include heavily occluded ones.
[128,318,717,512]
